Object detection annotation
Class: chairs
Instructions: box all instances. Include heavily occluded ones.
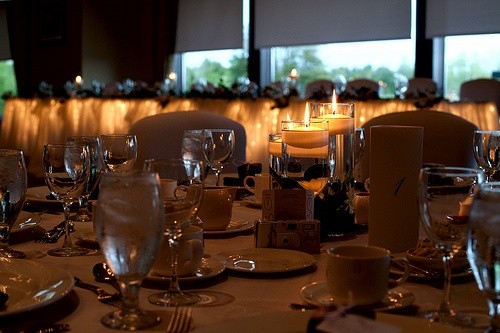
[128,78,500,184]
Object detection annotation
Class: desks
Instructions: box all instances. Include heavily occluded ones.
[0,170,494,333]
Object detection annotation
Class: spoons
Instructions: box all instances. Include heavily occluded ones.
[92,262,121,292]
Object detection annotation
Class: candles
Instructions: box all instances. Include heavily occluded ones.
[267,114,293,153]
[281,101,330,159]
[312,87,354,135]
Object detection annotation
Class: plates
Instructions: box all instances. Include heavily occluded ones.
[0,257,75,316]
[389,252,472,279]
[299,280,416,312]
[144,254,224,282]
[214,248,315,273]
[11,211,42,231]
[22,186,63,202]
[242,195,263,206]
[204,218,255,234]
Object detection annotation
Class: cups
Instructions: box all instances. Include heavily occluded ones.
[150,225,203,276]
[160,178,177,200]
[244,173,273,202]
[263,101,366,224]
[172,183,236,230]
[324,243,390,306]
[471,129,499,186]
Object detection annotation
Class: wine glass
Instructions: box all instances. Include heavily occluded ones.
[140,128,236,306]
[0,133,136,257]
[467,182,499,333]
[417,166,485,329]
[92,171,165,330]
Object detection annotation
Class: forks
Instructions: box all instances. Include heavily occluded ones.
[164,301,192,333]
[73,275,121,303]
[33,220,65,244]
